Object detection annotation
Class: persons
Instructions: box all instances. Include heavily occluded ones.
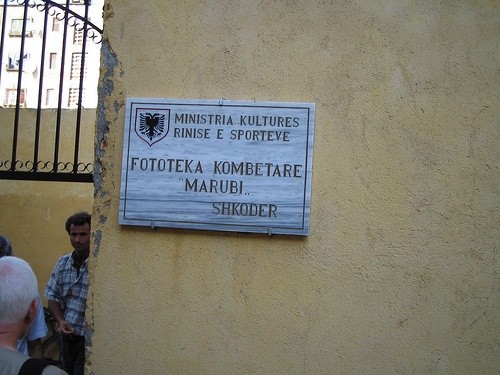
[0,211,90,375]
